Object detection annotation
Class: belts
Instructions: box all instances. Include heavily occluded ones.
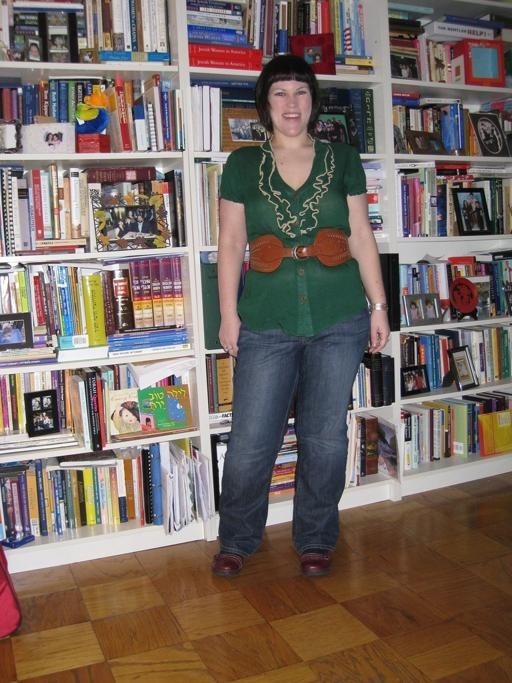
[247,228,352,275]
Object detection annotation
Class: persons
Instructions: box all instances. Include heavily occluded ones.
[211,54,391,576]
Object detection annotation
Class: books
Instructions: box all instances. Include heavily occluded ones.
[3,1,512,548]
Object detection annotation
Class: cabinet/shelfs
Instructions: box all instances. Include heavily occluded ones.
[0,0,512,574]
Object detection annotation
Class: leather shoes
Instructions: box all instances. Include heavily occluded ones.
[212,549,248,576]
[300,547,332,576]
